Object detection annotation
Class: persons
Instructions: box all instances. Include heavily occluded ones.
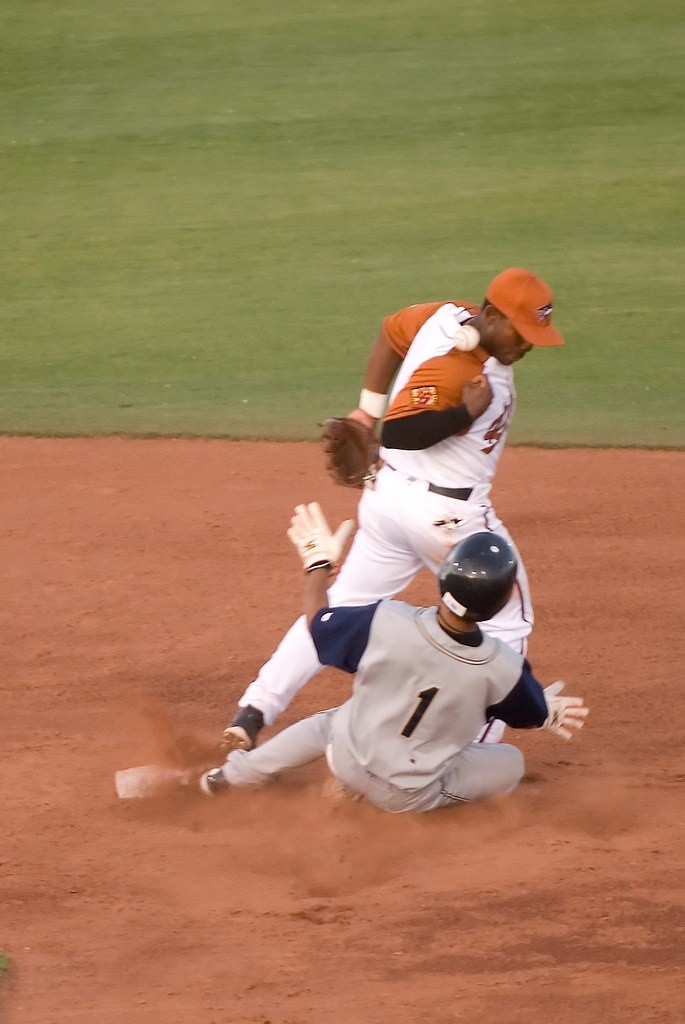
[200,500,591,811]
[220,268,564,749]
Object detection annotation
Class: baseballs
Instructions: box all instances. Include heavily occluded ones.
[453,324,481,353]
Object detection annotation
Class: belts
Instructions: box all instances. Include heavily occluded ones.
[384,461,472,501]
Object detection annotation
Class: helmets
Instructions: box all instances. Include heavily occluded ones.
[439,532,516,621]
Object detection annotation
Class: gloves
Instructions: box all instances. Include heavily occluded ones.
[287,502,354,572]
[535,681,589,739]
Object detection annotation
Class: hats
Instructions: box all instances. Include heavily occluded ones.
[486,267,565,347]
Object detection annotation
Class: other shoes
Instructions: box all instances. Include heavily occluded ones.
[200,768,229,798]
[221,704,264,752]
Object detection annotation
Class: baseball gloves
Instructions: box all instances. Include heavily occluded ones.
[317,411,383,492]
